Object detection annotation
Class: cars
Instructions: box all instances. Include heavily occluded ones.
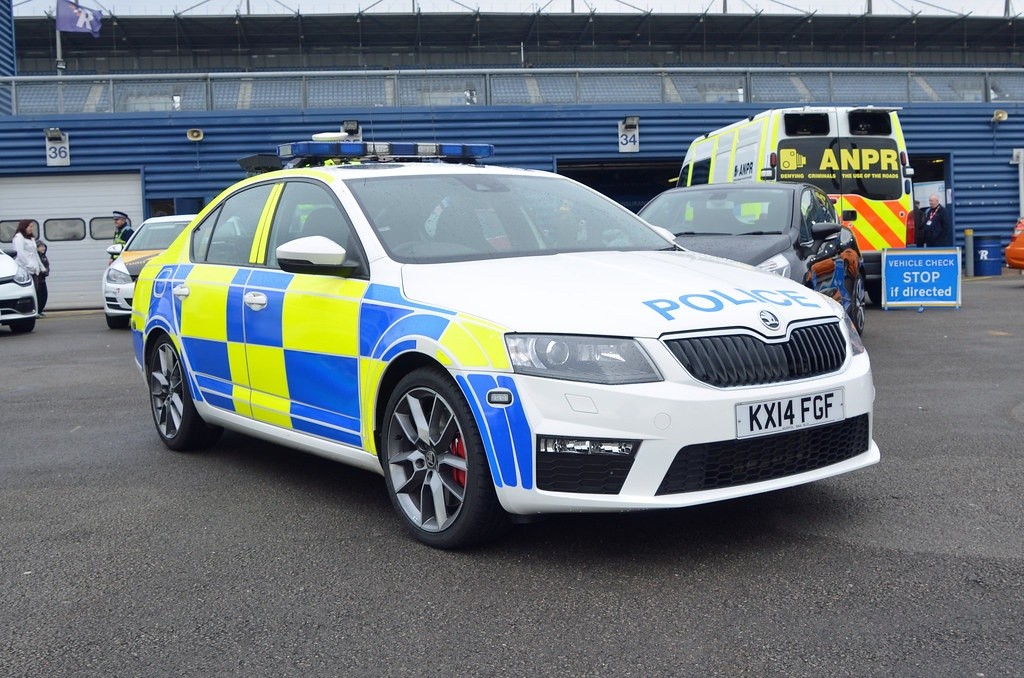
[101,213,201,331]
[0,248,38,335]
[132,141,883,550]
[635,180,866,335]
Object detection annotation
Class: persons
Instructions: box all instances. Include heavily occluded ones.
[923,195,951,248]
[913,200,927,247]
[33,240,52,319]
[10,219,48,297]
[107,211,135,266]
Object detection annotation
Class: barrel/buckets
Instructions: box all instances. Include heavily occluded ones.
[973,234,1003,276]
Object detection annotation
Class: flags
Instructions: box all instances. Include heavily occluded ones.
[55,0,103,39]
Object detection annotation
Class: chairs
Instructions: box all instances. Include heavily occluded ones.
[296,204,357,268]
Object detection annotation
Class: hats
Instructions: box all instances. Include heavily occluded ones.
[113,211,127,220]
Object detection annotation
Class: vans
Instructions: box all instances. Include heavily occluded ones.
[675,105,918,308]
